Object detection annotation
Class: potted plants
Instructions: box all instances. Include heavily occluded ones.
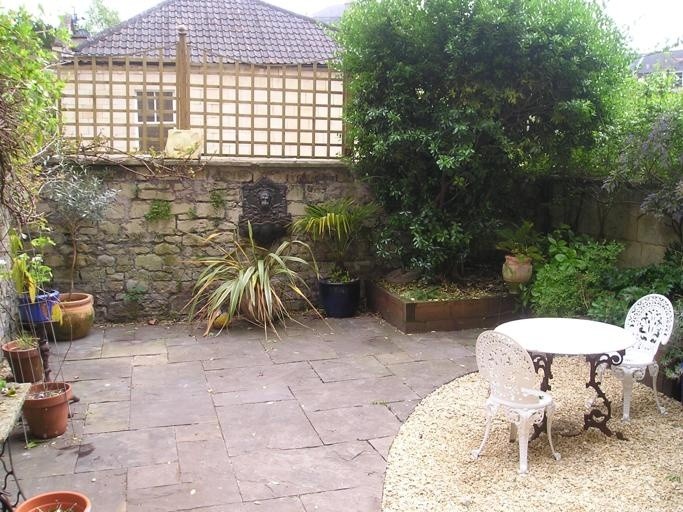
[10,230,66,326]
[492,219,537,290]
[291,192,380,317]
[171,228,335,344]
[30,158,115,345]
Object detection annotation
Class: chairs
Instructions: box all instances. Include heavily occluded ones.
[584,291,676,421]
[468,329,562,473]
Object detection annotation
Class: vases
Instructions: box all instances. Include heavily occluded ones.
[12,492,93,512]
[1,331,49,383]
[18,377,73,439]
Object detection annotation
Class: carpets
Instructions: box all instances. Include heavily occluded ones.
[378,353,681,512]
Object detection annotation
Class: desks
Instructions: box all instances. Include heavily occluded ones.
[0,377,37,512]
[491,316,636,449]
